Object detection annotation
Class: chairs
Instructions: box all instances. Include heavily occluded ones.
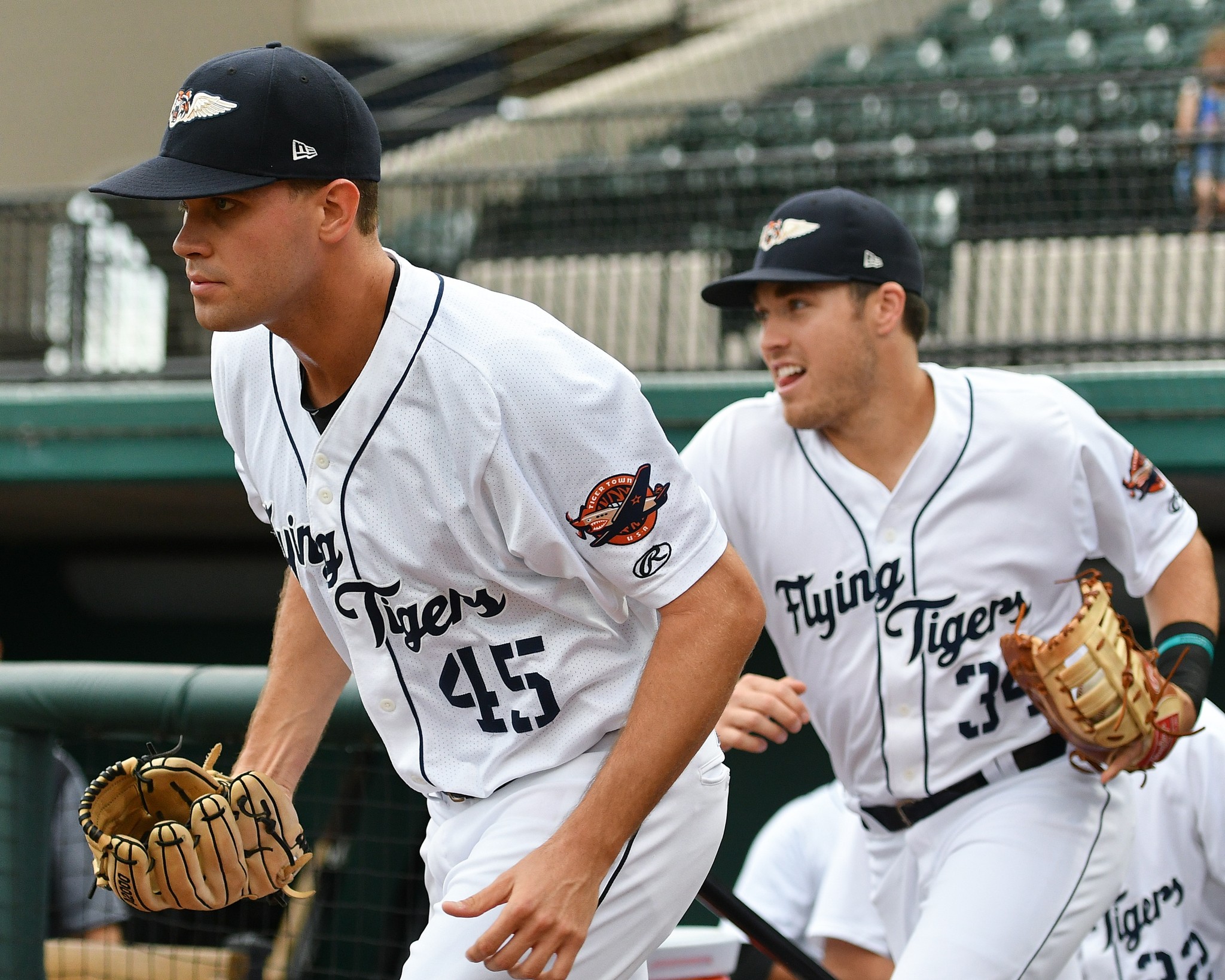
[473,0,1225,259]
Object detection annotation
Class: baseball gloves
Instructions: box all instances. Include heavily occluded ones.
[998,567,1207,790]
[77,734,317,912]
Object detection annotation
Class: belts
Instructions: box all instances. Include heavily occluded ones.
[862,732,1066,838]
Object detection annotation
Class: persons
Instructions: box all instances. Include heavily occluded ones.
[87,40,766,980]
[44,731,129,944]
[1173,32,1225,232]
[661,190,1220,980]
[736,665,1222,980]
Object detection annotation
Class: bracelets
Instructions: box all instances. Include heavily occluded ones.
[1154,632,1214,664]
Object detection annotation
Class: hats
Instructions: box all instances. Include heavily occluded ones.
[92,36,381,207]
[699,190,928,309]
